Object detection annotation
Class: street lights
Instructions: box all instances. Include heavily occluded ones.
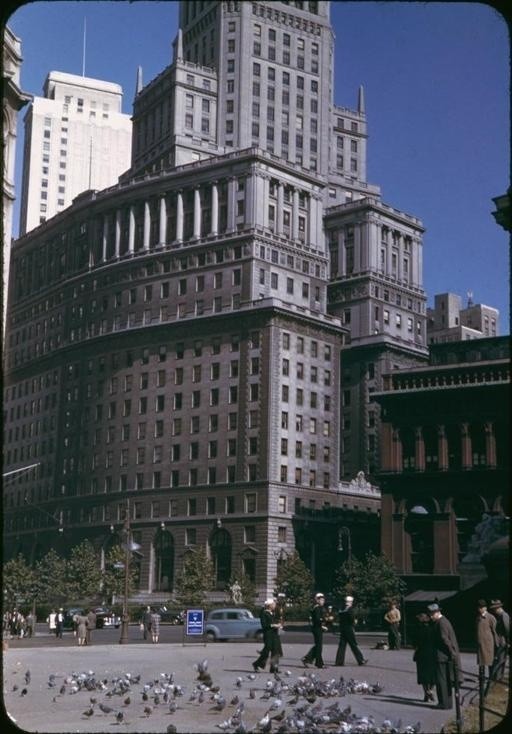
[336,526,353,576]
[118,496,141,645]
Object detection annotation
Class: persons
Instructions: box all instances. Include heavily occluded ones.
[143,606,161,644]
[301,593,368,669]
[46,608,65,639]
[476,600,509,676]
[384,600,402,650]
[73,607,97,646]
[3,608,37,640]
[252,597,283,673]
[231,581,244,605]
[413,604,459,709]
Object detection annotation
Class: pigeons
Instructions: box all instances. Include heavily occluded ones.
[13,684,18,691]
[25,676,30,683]
[188,660,422,734]
[25,670,31,677]
[20,688,28,698]
[46,670,185,724]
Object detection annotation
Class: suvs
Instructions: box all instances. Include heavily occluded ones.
[139,604,187,627]
[206,607,284,641]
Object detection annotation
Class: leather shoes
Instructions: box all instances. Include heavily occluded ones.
[252,662,282,673]
[430,704,453,710]
[336,658,370,666]
[301,656,327,669]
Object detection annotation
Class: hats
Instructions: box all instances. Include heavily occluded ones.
[415,603,443,617]
[265,598,274,606]
[345,595,354,602]
[475,599,504,608]
[315,592,324,602]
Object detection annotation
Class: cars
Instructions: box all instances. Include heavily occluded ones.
[62,606,121,629]
